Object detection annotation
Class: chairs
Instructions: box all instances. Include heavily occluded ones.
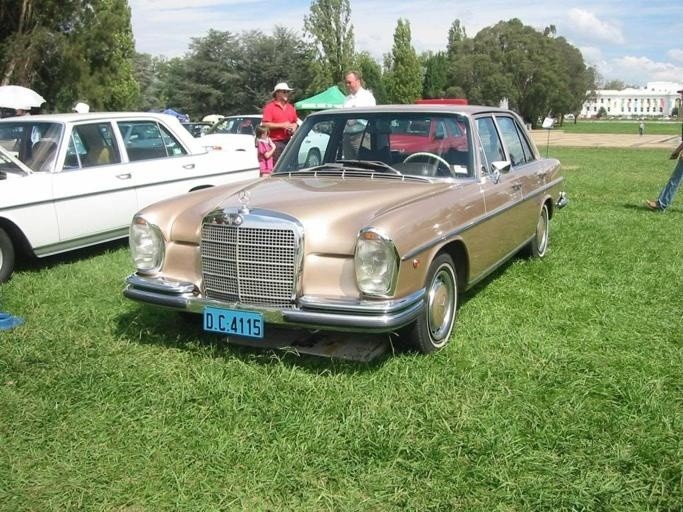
[80,131,115,167]
[19,136,58,176]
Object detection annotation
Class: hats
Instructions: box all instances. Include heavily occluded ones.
[72,103,90,113]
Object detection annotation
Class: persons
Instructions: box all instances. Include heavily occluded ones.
[344,71,376,160]
[639,121,644,134]
[15,109,41,152]
[255,125,276,176]
[261,82,298,167]
[645,88,683,209]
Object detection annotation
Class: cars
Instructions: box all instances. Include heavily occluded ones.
[86,101,328,184]
[386,96,471,154]
[607,114,648,120]
[0,109,263,290]
[672,114,677,120]
[656,114,671,120]
[565,114,575,120]
[114,96,573,355]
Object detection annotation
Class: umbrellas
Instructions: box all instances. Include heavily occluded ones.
[0,85,46,110]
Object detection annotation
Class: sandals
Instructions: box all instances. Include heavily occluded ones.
[645,198,665,213]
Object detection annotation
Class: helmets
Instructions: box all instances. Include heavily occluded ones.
[271,82,294,98]
